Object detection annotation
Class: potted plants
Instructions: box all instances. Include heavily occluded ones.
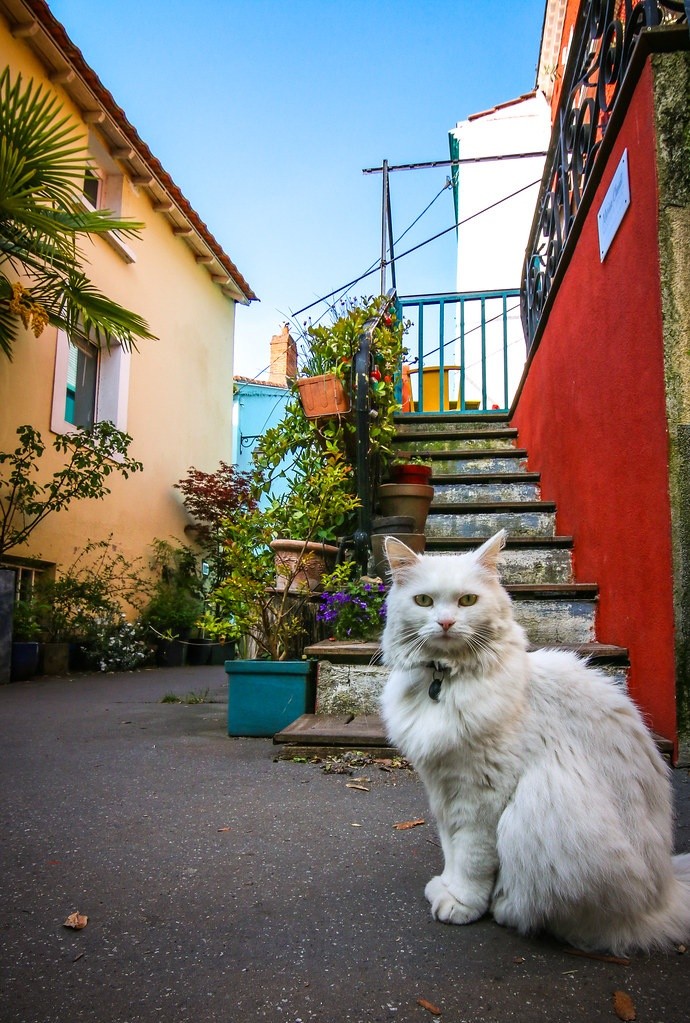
[388,456,433,485]
[0,294,417,737]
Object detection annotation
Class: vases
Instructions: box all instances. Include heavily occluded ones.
[378,483,435,536]
[371,533,427,585]
[371,516,416,534]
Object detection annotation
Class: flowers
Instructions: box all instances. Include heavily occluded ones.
[80,604,154,673]
[315,584,390,644]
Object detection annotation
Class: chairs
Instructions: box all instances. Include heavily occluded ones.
[404,366,463,412]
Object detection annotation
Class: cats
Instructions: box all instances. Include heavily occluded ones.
[375,527,690,957]
